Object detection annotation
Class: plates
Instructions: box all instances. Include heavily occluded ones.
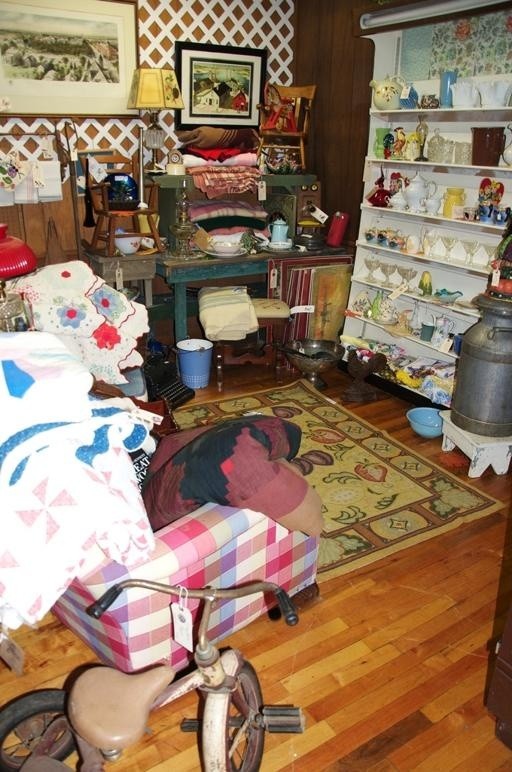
[196,215,326,258]
[104,174,138,200]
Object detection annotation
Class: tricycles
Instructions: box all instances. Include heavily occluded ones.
[0,577,310,772]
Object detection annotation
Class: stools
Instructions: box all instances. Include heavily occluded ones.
[213,298,290,393]
[84,252,161,307]
[438,410,512,479]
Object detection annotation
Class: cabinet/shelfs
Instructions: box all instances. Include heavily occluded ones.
[341,106,512,408]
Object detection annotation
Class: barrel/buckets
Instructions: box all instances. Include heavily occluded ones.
[451,292,512,437]
[176,338,214,390]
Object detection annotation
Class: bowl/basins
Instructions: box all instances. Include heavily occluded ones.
[112,234,144,255]
[406,406,444,440]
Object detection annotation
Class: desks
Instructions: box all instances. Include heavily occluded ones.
[157,241,355,377]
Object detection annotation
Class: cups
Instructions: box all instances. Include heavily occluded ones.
[363,69,512,347]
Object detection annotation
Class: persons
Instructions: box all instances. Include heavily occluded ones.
[0,27,118,83]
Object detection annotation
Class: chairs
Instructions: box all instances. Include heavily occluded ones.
[80,155,165,258]
[256,81,316,172]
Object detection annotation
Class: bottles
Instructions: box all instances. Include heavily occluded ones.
[327,204,352,247]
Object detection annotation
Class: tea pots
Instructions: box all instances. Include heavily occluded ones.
[264,158,299,175]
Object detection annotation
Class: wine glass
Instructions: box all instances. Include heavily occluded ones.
[283,339,345,391]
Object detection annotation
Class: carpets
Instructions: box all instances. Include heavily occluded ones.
[171,379,507,584]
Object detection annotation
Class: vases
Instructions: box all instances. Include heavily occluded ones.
[413,115,429,160]
[439,67,458,105]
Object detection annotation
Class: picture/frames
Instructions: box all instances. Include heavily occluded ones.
[175,41,267,131]
[0,0,141,118]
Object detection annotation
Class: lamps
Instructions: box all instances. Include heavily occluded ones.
[126,68,185,175]
[0,223,37,332]
